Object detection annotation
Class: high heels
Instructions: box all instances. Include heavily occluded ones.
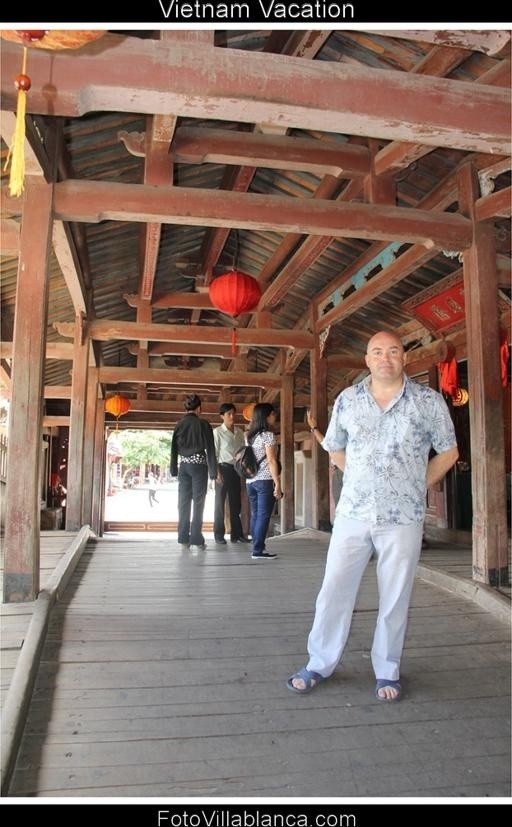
[178,542,207,552]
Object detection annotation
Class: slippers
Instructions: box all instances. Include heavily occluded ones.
[286,666,324,694]
[375,679,401,702]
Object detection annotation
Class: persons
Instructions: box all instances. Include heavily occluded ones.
[305,408,344,507]
[285,330,460,702]
[213,402,251,544]
[243,401,283,559]
[148,472,162,506]
[170,393,218,551]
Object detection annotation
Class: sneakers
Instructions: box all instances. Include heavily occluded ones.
[252,552,277,559]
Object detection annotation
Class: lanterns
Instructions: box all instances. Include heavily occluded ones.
[105,392,131,430]
[209,269,261,353]
[0,28,107,196]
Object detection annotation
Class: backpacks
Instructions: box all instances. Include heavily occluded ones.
[233,430,264,478]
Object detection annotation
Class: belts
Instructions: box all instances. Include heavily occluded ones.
[221,463,228,467]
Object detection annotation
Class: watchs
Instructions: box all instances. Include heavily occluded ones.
[310,426,318,432]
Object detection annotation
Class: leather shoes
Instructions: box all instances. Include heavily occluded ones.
[215,537,226,544]
[231,536,252,543]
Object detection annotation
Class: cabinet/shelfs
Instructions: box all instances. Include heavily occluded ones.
[41,506,63,530]
